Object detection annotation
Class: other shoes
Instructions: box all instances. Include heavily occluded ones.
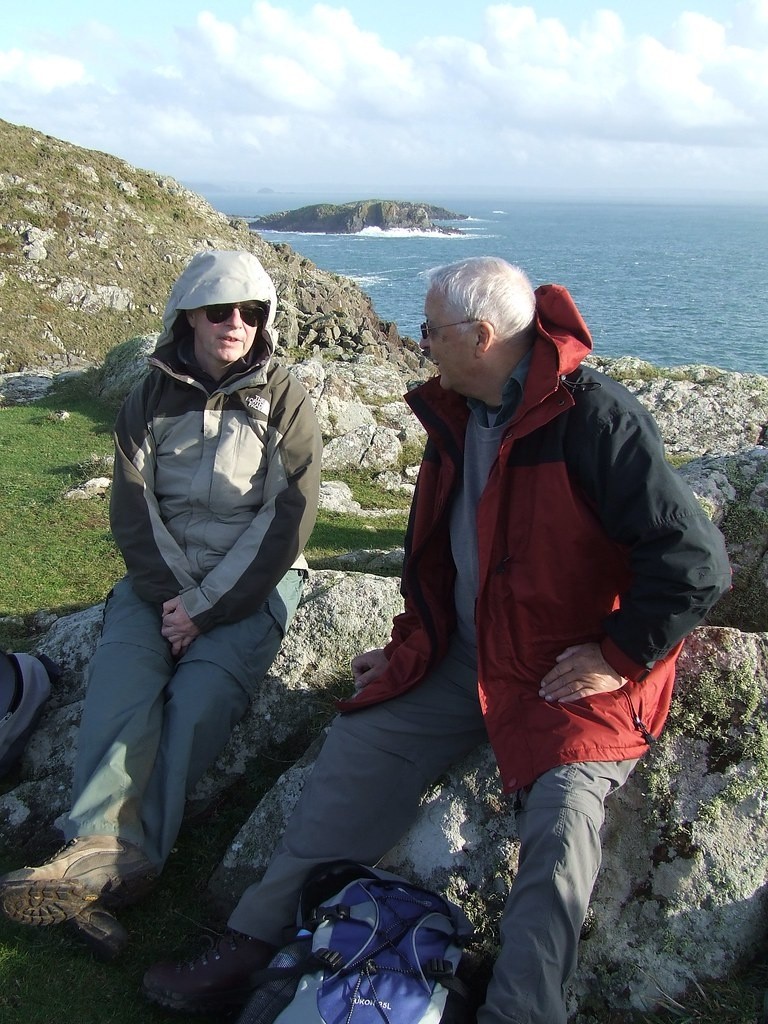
[141,928,280,1010]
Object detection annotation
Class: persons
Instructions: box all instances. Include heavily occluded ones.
[149,252,731,1024]
[1,249,321,963]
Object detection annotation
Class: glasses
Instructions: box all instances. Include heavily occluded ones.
[201,304,266,327]
[421,318,484,340]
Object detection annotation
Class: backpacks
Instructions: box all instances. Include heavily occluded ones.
[236,858,477,1024]
[0,650,62,784]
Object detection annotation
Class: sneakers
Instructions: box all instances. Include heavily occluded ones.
[0,835,158,966]
